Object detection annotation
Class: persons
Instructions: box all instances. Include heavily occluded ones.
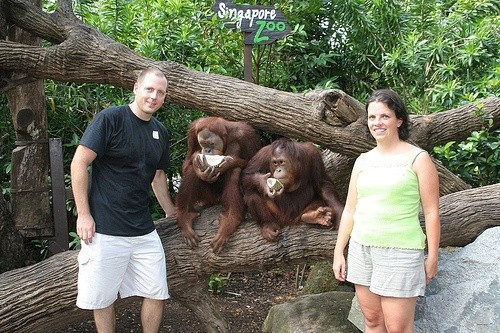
[331,89,441,333]
[70,68,179,333]
